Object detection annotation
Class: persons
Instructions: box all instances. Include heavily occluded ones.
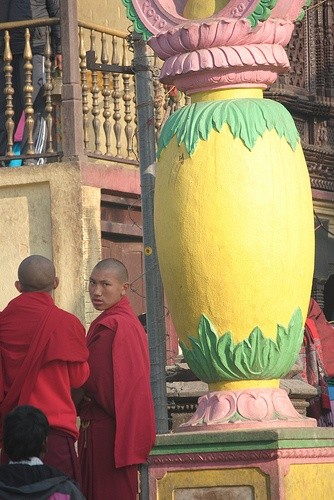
[0,255,89,490]
[291,297,334,430]
[0,405,88,500]
[86,258,156,500]
[0,1,62,167]
